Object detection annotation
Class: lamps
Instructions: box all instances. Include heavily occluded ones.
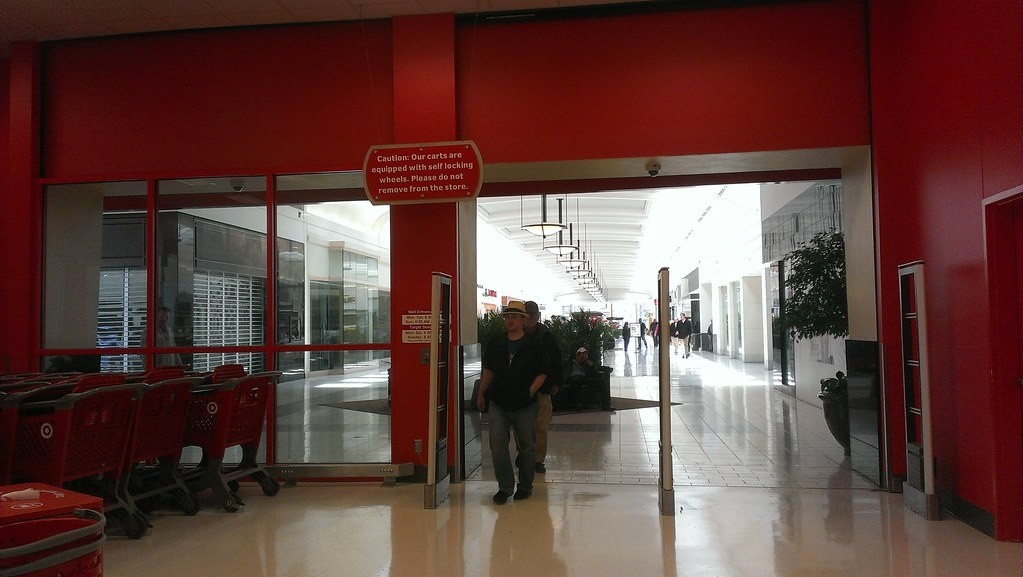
[520,195,608,303]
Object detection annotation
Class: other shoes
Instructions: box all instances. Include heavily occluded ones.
[602,405,616,411]
[535,462,547,473]
[493,490,511,505]
[513,490,532,500]
[515,454,521,468]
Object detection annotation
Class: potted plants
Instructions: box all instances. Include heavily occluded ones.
[774,227,851,457]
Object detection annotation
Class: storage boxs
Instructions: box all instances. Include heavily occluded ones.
[0,482,107,577]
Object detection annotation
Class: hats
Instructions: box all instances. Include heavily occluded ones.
[524,301,538,314]
[577,347,589,354]
[499,301,530,318]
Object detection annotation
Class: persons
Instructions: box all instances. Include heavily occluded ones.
[670,316,681,354]
[139,307,183,369]
[708,319,713,351]
[476,301,550,504]
[571,348,615,411]
[514,301,561,473]
[675,313,691,358]
[637,318,648,348]
[622,322,631,351]
[649,319,659,348]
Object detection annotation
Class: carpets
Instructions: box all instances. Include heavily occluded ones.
[319,399,683,423]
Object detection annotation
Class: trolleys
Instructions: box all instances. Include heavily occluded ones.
[0,362,284,542]
